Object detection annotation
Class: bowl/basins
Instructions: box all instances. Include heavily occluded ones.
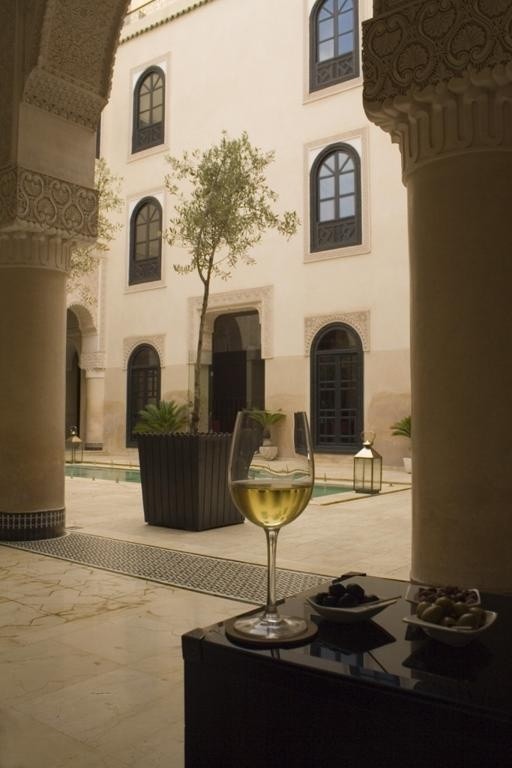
[306,584,497,649]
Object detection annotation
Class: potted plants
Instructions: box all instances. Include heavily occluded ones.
[131,126,305,531]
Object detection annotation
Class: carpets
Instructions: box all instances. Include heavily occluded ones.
[1,527,340,608]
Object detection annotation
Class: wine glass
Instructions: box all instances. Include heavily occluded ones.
[224,411,318,650]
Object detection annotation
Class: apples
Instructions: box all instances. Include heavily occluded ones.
[315,583,365,607]
[416,597,486,629]
